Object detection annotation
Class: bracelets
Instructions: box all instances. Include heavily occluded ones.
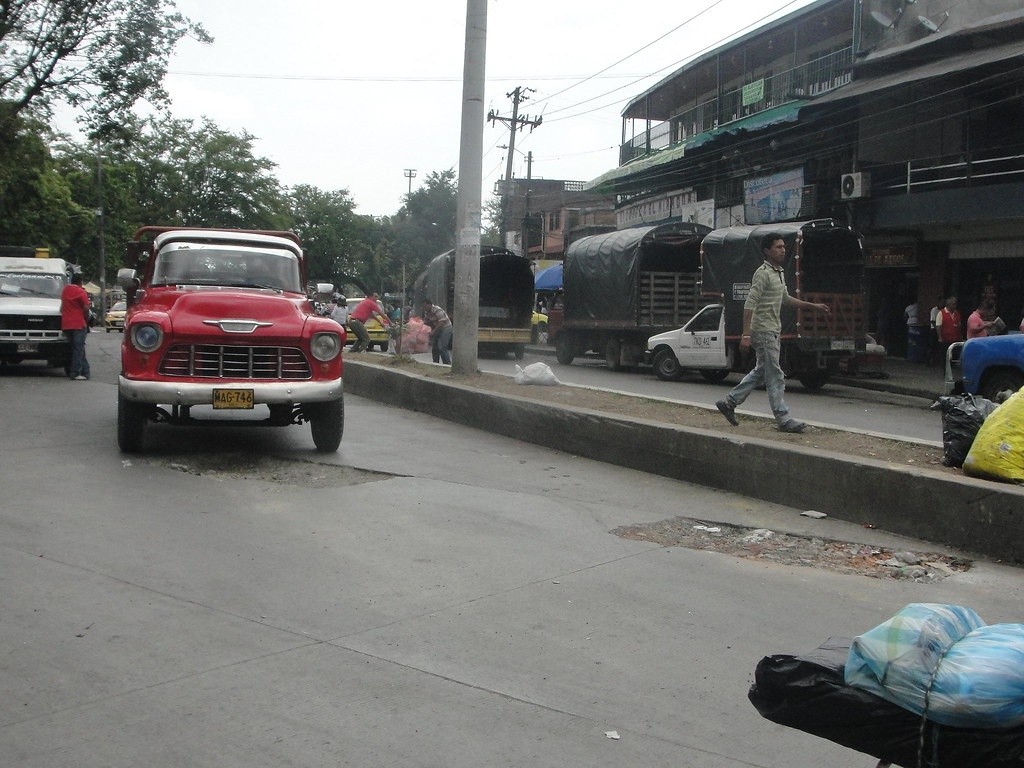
[743,335,751,338]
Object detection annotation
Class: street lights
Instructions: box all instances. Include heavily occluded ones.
[496,144,535,258]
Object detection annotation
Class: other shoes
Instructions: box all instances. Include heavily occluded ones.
[715,399,739,426]
[778,419,807,433]
[75,375,86,379]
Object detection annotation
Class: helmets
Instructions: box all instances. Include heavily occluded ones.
[332,292,340,300]
[337,295,346,305]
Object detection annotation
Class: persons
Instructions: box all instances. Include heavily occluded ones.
[390,304,412,324]
[322,296,347,329]
[60,275,90,380]
[716,233,830,431]
[905,296,996,377]
[348,293,393,353]
[421,299,452,365]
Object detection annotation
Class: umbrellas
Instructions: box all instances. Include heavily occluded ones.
[534,263,563,293]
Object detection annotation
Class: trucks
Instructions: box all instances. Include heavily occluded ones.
[548,217,868,391]
[116,227,346,453]
[414,244,535,359]
[945,333,1024,404]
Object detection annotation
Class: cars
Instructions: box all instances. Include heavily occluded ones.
[346,294,391,352]
[104,301,127,334]
[531,310,548,334]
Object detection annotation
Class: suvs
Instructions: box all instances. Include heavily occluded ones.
[0,257,80,375]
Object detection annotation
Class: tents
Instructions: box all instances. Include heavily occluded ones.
[82,281,124,307]
